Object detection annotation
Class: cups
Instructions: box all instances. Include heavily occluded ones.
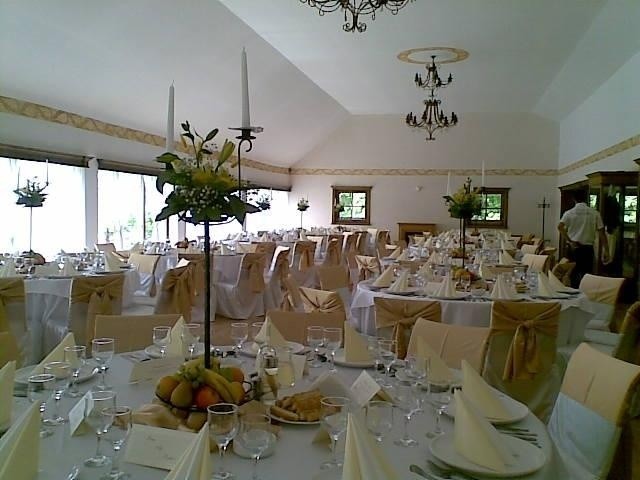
[365,401,393,448]
[229,322,248,348]
[252,320,269,348]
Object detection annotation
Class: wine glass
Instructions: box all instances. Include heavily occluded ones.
[391,356,452,448]
[63,345,87,400]
[84,391,117,469]
[92,338,114,390]
[368,334,395,391]
[393,263,538,303]
[100,406,134,480]
[307,325,323,369]
[43,360,68,429]
[206,404,239,480]
[154,326,172,360]
[27,375,55,441]
[320,397,351,475]
[322,330,341,372]
[181,322,201,358]
[239,415,271,478]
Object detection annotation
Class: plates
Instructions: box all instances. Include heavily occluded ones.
[263,401,337,426]
[394,365,463,392]
[241,340,304,356]
[427,431,545,476]
[441,397,531,428]
[15,361,100,385]
[370,277,580,301]
[327,347,392,369]
[148,342,215,358]
[395,369,470,388]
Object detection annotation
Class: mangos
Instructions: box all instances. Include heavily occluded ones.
[155,375,179,401]
[171,382,192,409]
[231,367,245,385]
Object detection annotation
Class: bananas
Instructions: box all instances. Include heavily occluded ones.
[204,367,239,405]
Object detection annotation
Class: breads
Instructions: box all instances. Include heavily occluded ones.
[270,387,339,422]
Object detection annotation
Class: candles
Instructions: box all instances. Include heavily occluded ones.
[17,168,19,188]
[241,47,250,128]
[447,170,451,196]
[166,80,175,152]
[46,158,49,182]
[481,161,484,188]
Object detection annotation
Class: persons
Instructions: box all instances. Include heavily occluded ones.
[558,187,610,290]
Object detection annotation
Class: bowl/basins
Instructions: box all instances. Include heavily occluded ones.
[43,362,71,379]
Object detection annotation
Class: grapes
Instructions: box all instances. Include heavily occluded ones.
[174,356,220,390]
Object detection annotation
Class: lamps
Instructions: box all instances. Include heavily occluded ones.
[405,55,458,141]
[299,1,413,33]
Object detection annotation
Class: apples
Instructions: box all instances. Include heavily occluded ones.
[195,385,219,410]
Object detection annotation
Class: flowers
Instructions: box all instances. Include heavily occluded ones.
[442,177,483,222]
[154,119,270,225]
[298,197,309,211]
[14,176,48,208]
